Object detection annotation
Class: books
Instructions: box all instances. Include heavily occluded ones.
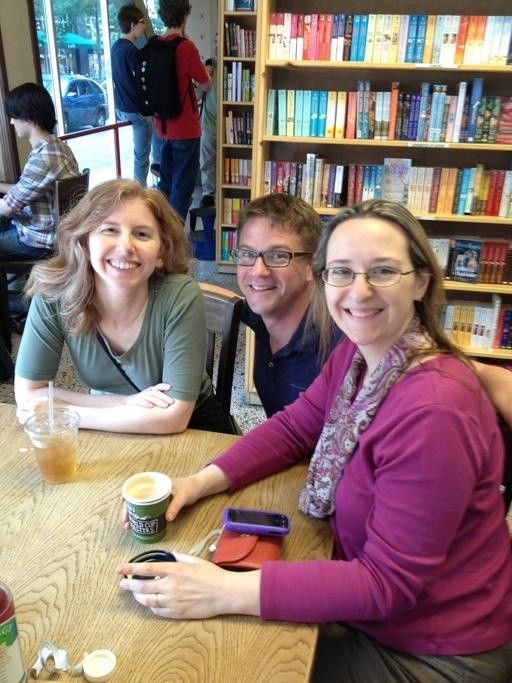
[424,236,510,351]
[264,152,512,217]
[219,109,254,261]
[265,77,512,145]
[222,1,256,102]
[268,11,512,67]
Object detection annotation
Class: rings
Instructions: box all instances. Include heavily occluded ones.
[155,594,160,608]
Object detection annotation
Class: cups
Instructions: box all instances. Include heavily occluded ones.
[23,408,81,484]
[121,471,173,544]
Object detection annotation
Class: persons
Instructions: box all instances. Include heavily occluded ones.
[9,175,240,439]
[193,30,221,208]
[115,196,512,683]
[146,0,211,223]
[230,192,512,433]
[107,3,166,190]
[0,81,82,387]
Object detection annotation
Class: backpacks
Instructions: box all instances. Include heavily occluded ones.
[132,40,184,122]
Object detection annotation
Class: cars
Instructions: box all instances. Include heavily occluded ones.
[42,74,107,133]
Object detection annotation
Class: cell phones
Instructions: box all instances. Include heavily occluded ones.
[222,506,291,538]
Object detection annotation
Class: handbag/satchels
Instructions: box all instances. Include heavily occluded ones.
[187,392,236,434]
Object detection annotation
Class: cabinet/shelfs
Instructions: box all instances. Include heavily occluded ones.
[252,5,512,358]
[214,2,261,281]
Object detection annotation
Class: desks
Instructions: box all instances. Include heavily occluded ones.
[1,402,334,683]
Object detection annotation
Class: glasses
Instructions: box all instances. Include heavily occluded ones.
[321,264,420,288]
[230,246,311,268]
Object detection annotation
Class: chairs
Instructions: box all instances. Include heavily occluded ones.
[0,168,89,354]
[194,282,245,409]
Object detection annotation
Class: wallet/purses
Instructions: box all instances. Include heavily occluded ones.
[209,525,288,572]
